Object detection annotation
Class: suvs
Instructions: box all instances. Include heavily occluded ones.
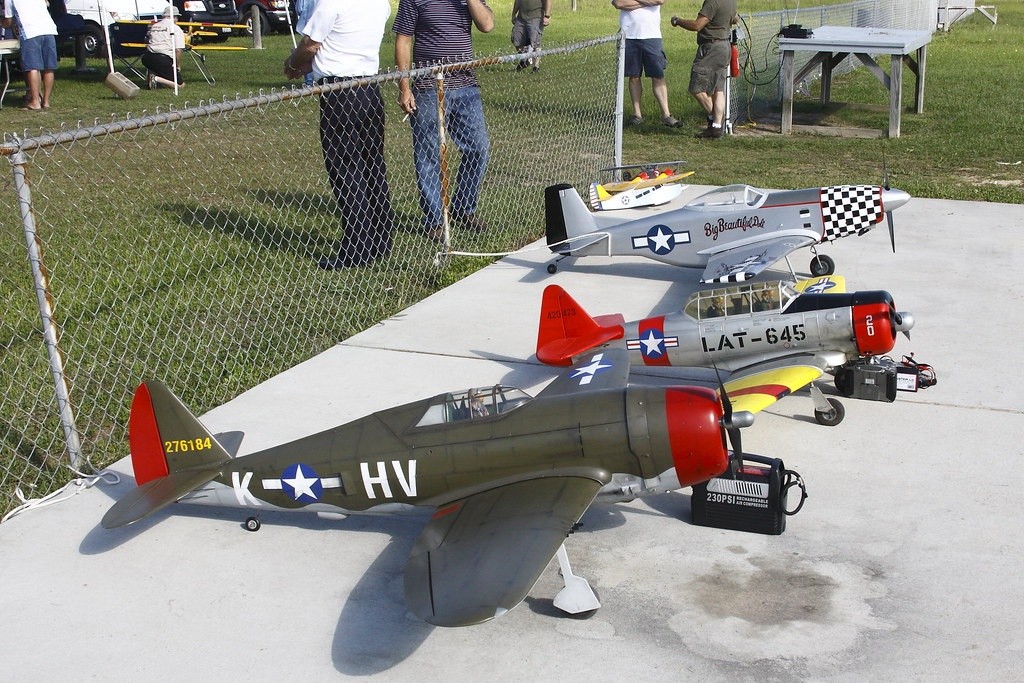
[0,0,302,57]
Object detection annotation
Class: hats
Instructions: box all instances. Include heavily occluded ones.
[161,6,182,18]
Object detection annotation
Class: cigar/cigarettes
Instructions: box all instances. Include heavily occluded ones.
[402,113,410,122]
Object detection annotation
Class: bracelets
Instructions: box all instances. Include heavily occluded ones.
[544,15,550,19]
[288,58,297,71]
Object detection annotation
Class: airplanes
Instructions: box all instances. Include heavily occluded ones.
[101,347,757,628]
[536,274,914,426]
[546,135,910,282]
[589,160,695,212]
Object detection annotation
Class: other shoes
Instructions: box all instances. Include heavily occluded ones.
[457,216,487,230]
[516,60,530,71]
[319,247,392,271]
[695,127,722,138]
[708,119,713,127]
[424,224,444,241]
[629,116,645,125]
[147,74,154,89]
[532,64,540,74]
[664,117,682,127]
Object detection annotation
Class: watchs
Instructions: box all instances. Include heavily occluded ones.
[674,18,678,25]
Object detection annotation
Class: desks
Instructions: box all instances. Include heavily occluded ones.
[778,26,932,140]
[938,5,998,31]
[0,49,44,110]
[0,185,1024,683]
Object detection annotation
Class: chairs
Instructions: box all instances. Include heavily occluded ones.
[109,23,147,81]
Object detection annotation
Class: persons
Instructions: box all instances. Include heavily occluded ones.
[612,0,683,129]
[708,296,726,317]
[142,6,185,90]
[468,388,489,418]
[511,0,551,75]
[671,0,739,139]
[2,0,58,110]
[284,0,393,269]
[295,0,319,89]
[392,0,494,245]
[761,289,776,310]
